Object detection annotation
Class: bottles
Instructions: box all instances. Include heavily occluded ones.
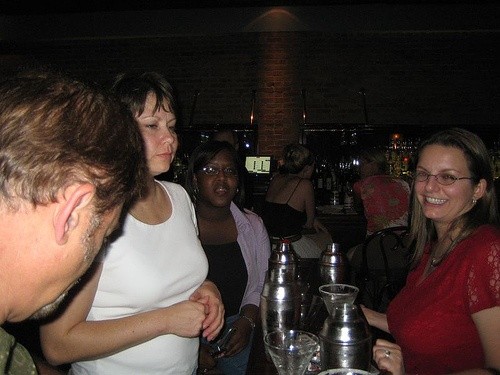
[318,305,372,372]
[311,158,354,214]
[261,236,301,362]
[317,243,350,284]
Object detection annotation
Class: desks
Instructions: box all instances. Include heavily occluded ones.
[314,200,367,242]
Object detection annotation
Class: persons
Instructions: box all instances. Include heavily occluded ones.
[0,63,153,375]
[359,125,500,375]
[256,141,335,258]
[344,151,412,308]
[39,70,228,375]
[187,141,274,375]
[212,128,240,152]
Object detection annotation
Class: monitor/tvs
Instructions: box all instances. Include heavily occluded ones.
[244,153,273,175]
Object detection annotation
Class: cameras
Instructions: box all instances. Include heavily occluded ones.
[200,326,236,353]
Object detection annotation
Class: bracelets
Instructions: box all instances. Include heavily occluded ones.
[239,313,255,331]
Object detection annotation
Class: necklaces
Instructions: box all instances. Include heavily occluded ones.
[429,231,466,270]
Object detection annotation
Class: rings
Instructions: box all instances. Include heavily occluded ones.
[384,350,392,358]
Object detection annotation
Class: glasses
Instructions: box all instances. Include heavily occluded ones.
[412,169,473,187]
[200,165,238,177]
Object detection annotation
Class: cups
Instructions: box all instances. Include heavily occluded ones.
[263,329,319,375]
[319,284,359,314]
